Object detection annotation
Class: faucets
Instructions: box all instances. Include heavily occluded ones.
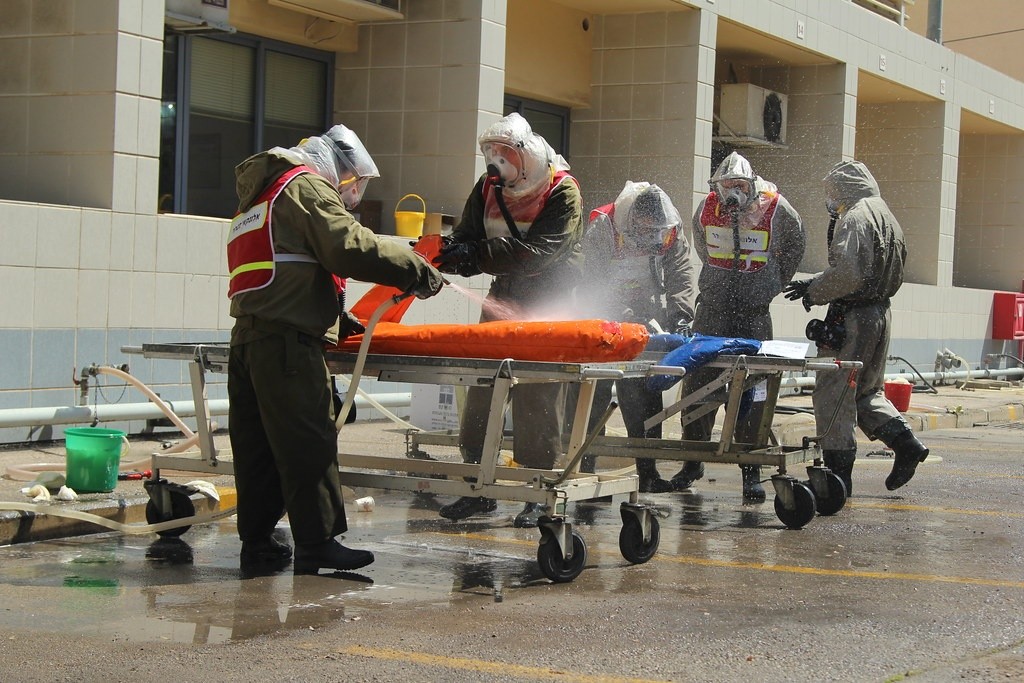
[72,365,92,389]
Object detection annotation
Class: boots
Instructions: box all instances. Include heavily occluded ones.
[886,429,930,490]
[823,448,857,496]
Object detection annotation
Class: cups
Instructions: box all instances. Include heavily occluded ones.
[354,496,375,512]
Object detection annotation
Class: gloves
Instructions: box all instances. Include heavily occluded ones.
[783,278,809,301]
[802,294,813,312]
[409,232,451,255]
[432,242,483,277]
[670,315,693,338]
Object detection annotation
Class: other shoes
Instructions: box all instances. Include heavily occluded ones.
[743,468,766,497]
[672,463,705,489]
[293,535,375,574]
[240,536,292,565]
[638,476,674,493]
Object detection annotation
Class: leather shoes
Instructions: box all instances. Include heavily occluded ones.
[514,501,547,525]
[439,495,496,518]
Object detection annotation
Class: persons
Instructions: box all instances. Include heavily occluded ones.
[783,159,930,498]
[409,112,584,528]
[669,151,805,499]
[579,180,695,502]
[226,124,443,575]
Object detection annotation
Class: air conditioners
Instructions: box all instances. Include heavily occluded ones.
[269,0,404,26]
[717,82,788,146]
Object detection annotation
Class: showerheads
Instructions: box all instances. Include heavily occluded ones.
[441,274,451,289]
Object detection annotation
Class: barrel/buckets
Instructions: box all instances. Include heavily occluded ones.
[394,194,426,238]
[64,428,129,491]
[884,382,913,412]
